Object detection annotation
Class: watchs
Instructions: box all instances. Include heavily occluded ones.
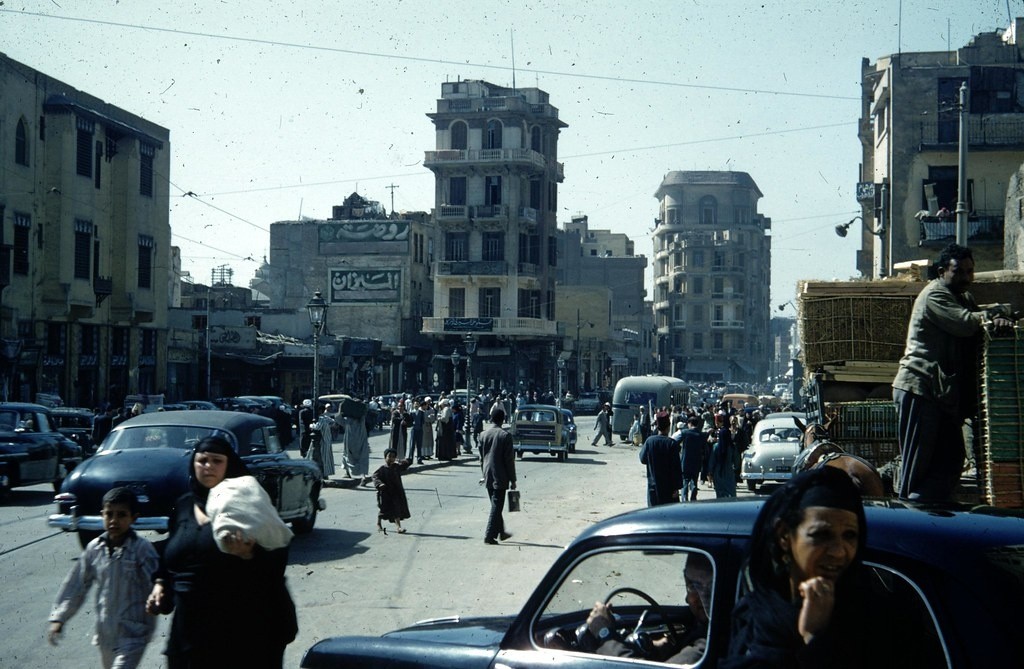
[597,627,616,641]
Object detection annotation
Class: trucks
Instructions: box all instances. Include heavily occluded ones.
[793,375,901,468]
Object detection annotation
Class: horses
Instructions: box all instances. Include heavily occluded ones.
[789,414,885,496]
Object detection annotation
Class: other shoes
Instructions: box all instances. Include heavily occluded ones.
[397,527,406,533]
[499,533,511,541]
[484,538,499,545]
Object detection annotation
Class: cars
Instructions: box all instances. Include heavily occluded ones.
[0,402,82,500]
[317,394,356,430]
[739,413,806,491]
[51,406,101,458]
[296,486,1023,669]
[158,395,297,438]
[48,411,326,551]
[507,405,578,461]
[378,390,480,411]
[697,381,794,409]
[576,390,611,413]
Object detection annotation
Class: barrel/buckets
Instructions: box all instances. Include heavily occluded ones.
[508,490,520,512]
[633,432,642,443]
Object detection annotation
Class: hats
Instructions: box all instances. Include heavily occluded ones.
[640,406,645,410]
[441,399,449,405]
[605,402,611,407]
[715,414,722,425]
[655,412,671,430]
[303,400,312,408]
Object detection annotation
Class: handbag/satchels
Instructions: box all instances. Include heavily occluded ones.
[633,432,642,445]
[436,419,443,437]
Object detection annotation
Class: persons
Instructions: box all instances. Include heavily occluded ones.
[371,448,414,534]
[477,409,517,544]
[583,552,713,666]
[891,243,1015,499]
[729,466,928,668]
[90,383,790,545]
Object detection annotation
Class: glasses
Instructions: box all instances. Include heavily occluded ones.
[684,577,711,602]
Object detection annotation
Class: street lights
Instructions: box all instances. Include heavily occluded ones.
[835,185,887,281]
[208,295,230,397]
[576,321,595,390]
[307,290,328,411]
[464,333,477,454]
[556,356,566,408]
[450,349,462,392]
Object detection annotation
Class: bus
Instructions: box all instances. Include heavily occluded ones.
[610,373,690,439]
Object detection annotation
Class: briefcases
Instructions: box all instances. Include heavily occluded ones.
[504,486,521,512]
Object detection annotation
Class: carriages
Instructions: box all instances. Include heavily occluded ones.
[789,414,1004,516]
